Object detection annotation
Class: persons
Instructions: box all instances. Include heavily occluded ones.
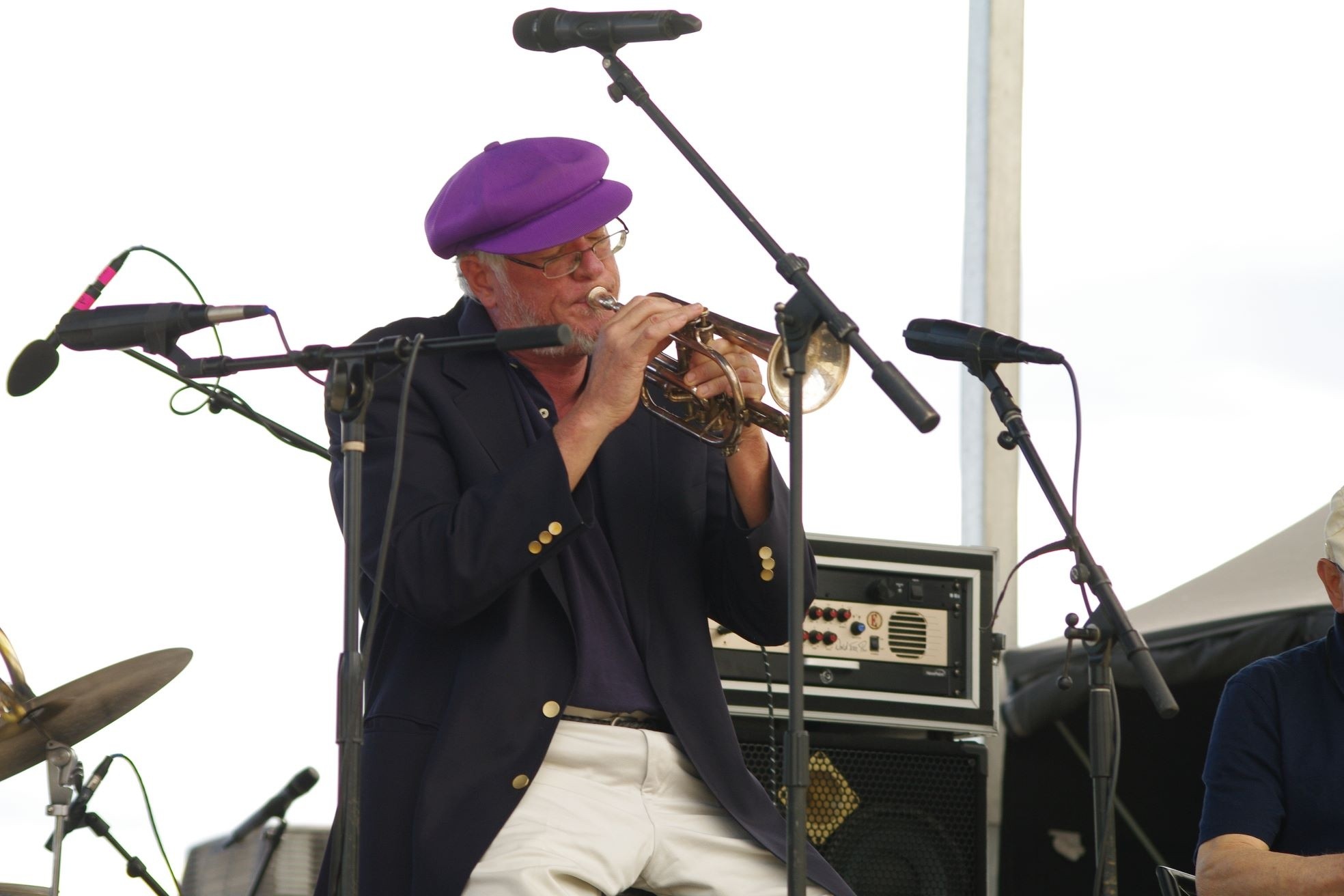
[1195,485,1344,896]
[324,135,860,895]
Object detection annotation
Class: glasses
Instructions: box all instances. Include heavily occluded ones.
[503,216,629,279]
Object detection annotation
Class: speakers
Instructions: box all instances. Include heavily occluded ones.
[725,705,1004,896]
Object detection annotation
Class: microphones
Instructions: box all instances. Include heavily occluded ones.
[7,250,130,397]
[225,767,319,849]
[901,319,1063,366]
[45,755,112,852]
[54,303,269,350]
[512,8,702,52]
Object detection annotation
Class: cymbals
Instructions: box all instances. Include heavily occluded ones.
[0,646,194,783]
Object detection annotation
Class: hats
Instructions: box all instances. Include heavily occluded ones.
[1324,486,1344,574]
[424,137,633,260]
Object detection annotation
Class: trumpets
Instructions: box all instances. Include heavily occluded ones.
[585,285,851,458]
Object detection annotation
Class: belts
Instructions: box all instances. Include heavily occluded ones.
[558,713,675,735]
